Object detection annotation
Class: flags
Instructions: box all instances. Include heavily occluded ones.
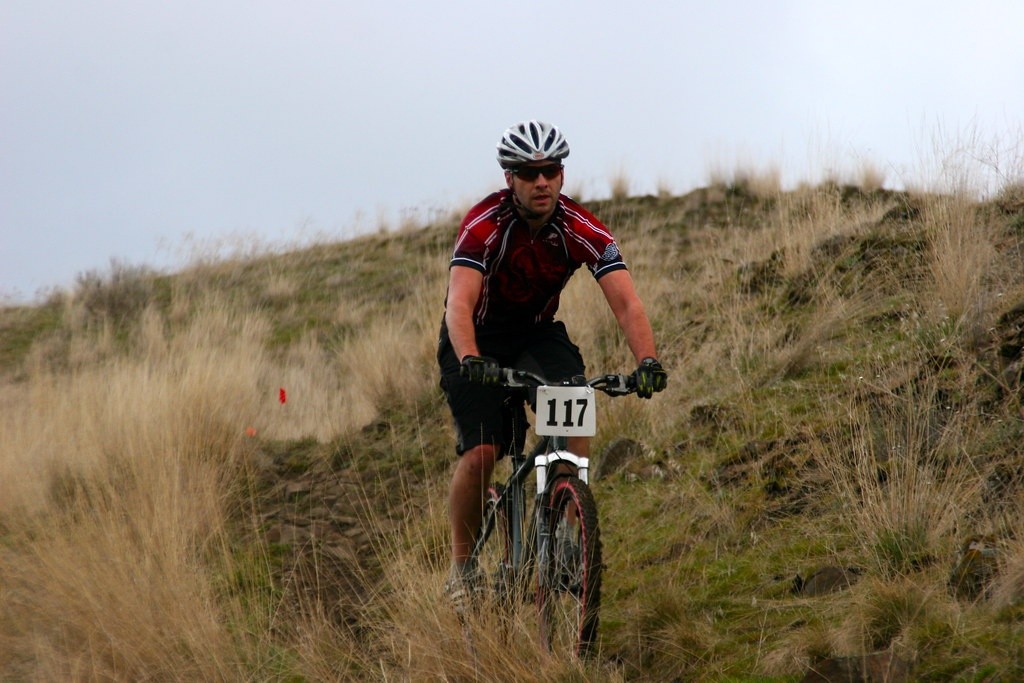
[279,386,287,404]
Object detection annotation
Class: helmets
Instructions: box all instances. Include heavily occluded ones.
[496,118,572,171]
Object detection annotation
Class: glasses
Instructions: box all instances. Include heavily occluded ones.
[512,163,562,182]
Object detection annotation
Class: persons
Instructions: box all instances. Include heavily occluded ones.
[433,120,667,619]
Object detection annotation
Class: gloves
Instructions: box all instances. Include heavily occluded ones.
[629,356,667,401]
[460,354,499,386]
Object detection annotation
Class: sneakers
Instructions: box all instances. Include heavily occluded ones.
[442,565,487,616]
[553,519,581,568]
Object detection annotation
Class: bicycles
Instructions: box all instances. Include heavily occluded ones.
[459,365,666,658]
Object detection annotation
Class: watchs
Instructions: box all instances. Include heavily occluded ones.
[639,357,664,370]
